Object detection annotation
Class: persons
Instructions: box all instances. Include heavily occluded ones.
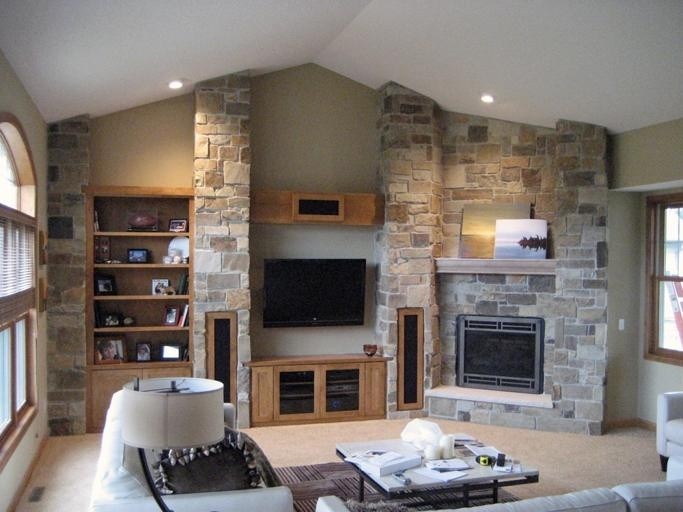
[137,343,151,361]
[97,340,122,360]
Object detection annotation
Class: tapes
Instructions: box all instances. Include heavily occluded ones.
[497,453,505,466]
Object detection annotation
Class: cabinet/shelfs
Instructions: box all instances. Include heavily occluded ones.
[243,354,394,427]
[82,185,194,433]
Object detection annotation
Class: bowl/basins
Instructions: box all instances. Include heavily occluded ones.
[363,344,377,356]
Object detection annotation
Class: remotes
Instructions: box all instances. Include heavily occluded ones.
[392,472,412,486]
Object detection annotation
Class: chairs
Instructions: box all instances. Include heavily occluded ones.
[656,391,683,471]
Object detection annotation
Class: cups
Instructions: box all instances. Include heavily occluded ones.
[424,435,454,459]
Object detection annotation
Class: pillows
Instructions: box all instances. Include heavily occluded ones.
[151,431,266,495]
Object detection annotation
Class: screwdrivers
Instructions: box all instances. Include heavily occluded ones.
[491,456,496,470]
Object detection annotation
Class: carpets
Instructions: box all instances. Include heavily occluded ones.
[274,461,521,512]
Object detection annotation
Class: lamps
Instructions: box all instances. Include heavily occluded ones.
[120,377,225,512]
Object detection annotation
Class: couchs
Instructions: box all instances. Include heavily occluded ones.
[89,390,294,512]
[314,457,683,512]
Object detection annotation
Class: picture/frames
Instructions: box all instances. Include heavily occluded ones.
[160,344,182,361]
[168,219,187,232]
[95,276,117,295]
[136,342,152,361]
[162,305,181,326]
[128,249,147,263]
[94,335,128,365]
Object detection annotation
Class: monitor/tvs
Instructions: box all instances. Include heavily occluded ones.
[262,257,367,327]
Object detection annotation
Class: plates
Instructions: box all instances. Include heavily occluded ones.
[168,237,189,257]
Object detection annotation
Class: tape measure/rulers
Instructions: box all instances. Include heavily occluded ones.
[475,455,492,466]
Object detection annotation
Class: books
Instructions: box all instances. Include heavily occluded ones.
[342,432,513,486]
[177,271,189,329]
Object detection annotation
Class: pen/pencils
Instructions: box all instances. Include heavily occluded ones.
[440,469,463,473]
